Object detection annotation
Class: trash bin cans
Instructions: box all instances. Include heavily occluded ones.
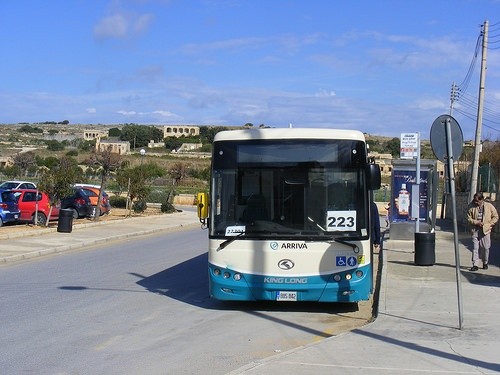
[414,231,436,267]
[57,208,75,233]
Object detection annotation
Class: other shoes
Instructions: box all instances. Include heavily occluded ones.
[483,264,488,269]
[471,266,478,270]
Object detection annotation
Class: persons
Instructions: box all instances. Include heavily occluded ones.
[466,192,499,271]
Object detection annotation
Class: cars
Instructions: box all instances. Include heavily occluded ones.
[0,181,37,193]
[61,184,110,218]
[12,189,60,225]
[0,190,21,226]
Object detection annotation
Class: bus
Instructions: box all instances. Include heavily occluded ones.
[198,121,381,305]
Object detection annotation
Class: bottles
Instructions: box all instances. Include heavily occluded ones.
[399,184,409,215]
